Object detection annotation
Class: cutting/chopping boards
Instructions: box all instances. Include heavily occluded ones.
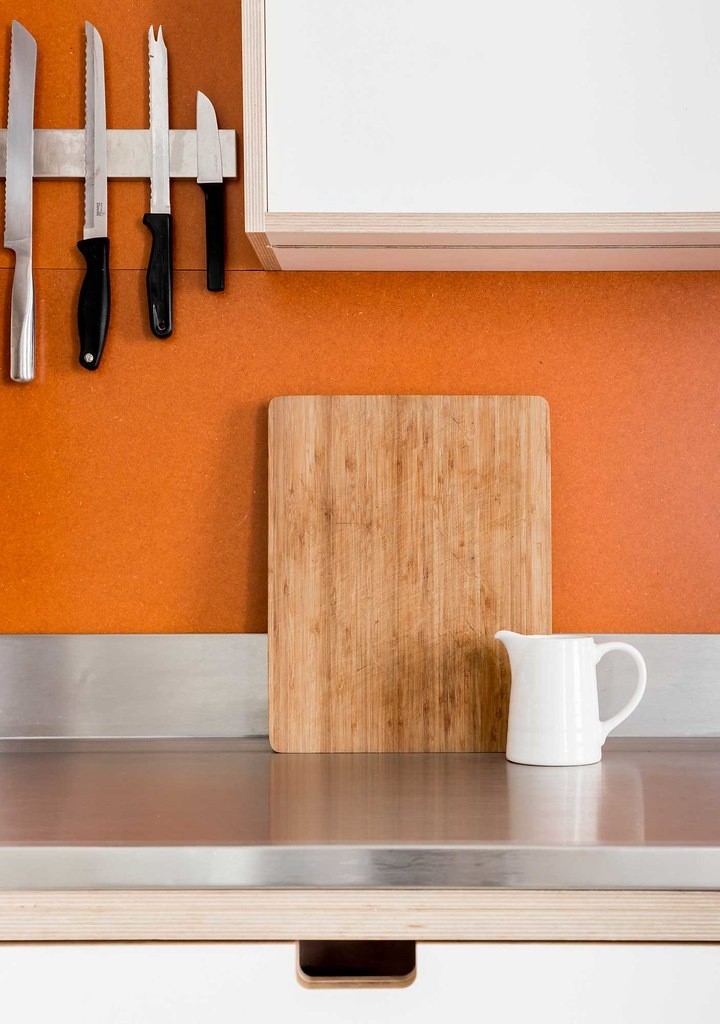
[267,394,553,754]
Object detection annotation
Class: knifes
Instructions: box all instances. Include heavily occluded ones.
[142,24,174,339]
[3,19,37,382]
[77,20,110,371]
[196,89,225,292]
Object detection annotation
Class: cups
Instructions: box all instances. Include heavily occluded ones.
[495,629,647,766]
